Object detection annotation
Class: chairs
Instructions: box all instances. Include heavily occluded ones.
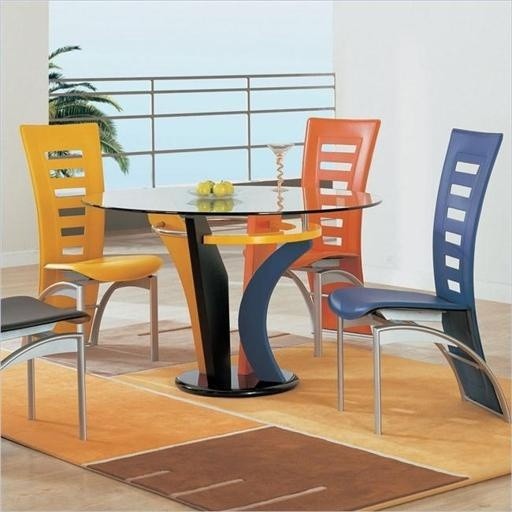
[0,296,92,440]
[241,117,381,355]
[19,121,163,362]
[328,129,512,436]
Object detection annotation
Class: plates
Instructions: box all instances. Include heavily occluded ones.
[187,198,245,206]
[187,188,245,199]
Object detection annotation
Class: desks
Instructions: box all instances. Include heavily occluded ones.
[82,185,335,399]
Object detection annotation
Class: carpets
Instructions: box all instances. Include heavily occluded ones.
[0,319,511,512]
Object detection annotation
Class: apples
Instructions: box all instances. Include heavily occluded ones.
[196,180,235,197]
[196,198,234,212]
